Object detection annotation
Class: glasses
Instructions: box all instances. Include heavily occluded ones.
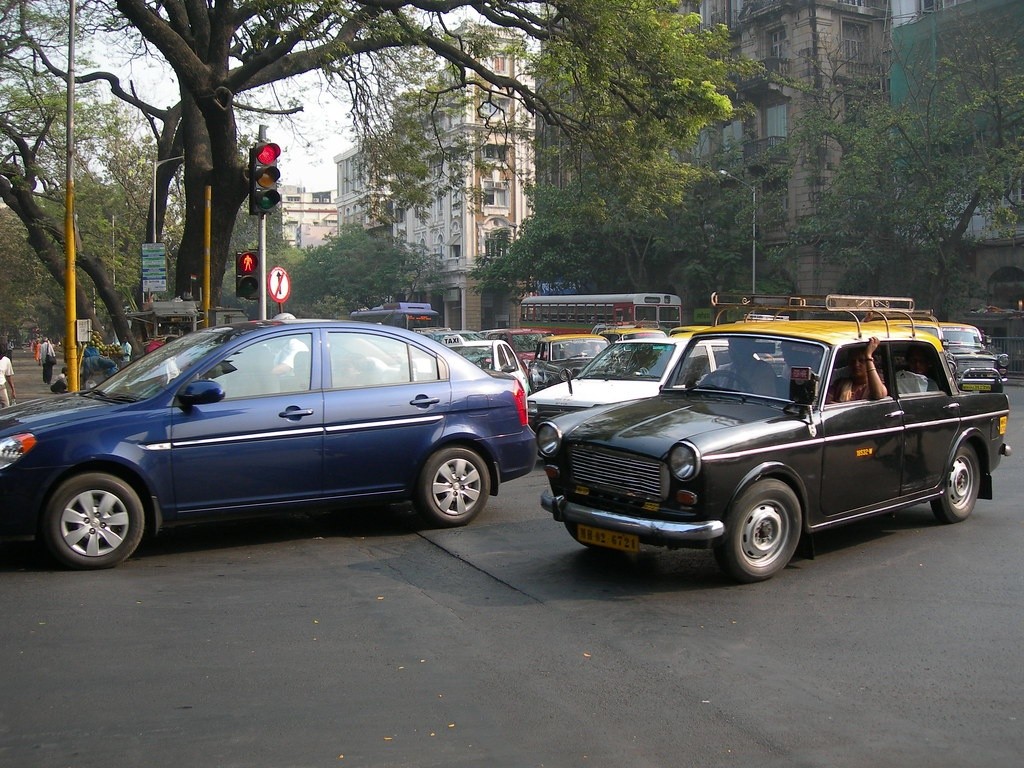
[850,357,866,363]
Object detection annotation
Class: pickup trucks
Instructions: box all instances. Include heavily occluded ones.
[535,292,1010,584]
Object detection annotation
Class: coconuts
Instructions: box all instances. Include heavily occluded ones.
[90,330,124,356]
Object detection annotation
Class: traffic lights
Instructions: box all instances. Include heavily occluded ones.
[249,142,281,216]
[236,249,260,300]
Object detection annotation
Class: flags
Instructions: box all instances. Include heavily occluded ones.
[191,275,199,281]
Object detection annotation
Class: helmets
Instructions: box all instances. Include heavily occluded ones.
[272,313,298,321]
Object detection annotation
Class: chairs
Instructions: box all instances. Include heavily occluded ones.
[293,351,311,391]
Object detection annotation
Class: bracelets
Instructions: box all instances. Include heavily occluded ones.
[866,358,874,361]
[867,368,876,371]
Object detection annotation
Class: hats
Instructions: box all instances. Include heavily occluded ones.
[57,374,65,380]
[35,338,41,341]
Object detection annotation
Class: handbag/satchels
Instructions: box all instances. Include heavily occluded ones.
[43,337,48,341]
[10,399,18,405]
[44,343,57,365]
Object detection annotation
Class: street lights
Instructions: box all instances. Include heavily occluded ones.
[718,170,755,315]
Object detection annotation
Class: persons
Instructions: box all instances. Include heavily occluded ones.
[824,336,887,404]
[696,337,776,398]
[905,345,939,391]
[35,339,41,366]
[0,343,16,408]
[40,336,56,384]
[50,367,68,394]
[121,336,132,362]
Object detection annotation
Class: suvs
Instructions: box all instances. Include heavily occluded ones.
[869,320,1010,393]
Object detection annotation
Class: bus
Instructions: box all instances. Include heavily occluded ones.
[520,294,681,336]
[350,302,439,331]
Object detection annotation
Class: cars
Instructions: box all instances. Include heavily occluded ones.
[412,323,714,368]
[527,337,691,434]
[441,334,531,399]
[527,333,611,396]
[0,319,537,571]
